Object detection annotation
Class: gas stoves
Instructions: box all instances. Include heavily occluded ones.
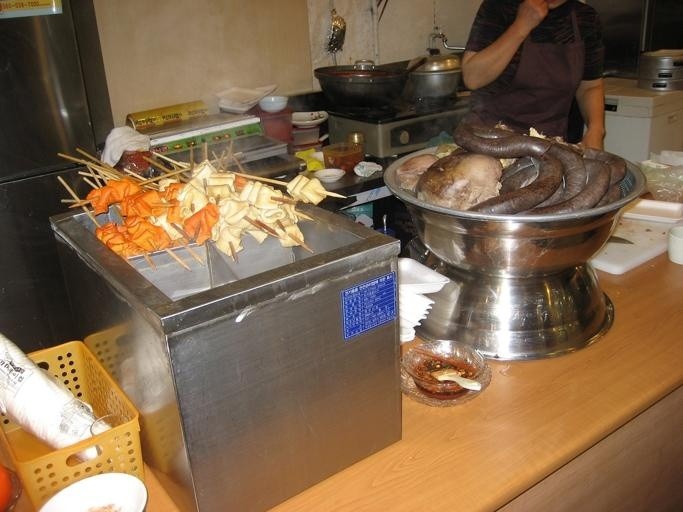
[293,89,473,159]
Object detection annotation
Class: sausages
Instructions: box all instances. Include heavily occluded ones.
[453,122,629,215]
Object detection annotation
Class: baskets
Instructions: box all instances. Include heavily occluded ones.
[78,321,181,473]
[0,341,143,511]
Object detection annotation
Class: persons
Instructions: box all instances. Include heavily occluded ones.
[457,0,609,154]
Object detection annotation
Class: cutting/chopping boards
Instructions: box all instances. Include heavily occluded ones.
[586,214,669,276]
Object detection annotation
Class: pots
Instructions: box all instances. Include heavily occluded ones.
[313,60,409,108]
[409,46,463,98]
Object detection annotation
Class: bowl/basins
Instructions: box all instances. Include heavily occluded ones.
[260,95,289,113]
[381,141,649,363]
[404,339,485,400]
[315,168,347,183]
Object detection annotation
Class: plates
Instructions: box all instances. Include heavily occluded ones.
[623,198,683,224]
[215,84,278,115]
[290,111,328,128]
[39,472,148,512]
[398,359,492,406]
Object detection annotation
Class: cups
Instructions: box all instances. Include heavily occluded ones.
[667,226,683,265]
